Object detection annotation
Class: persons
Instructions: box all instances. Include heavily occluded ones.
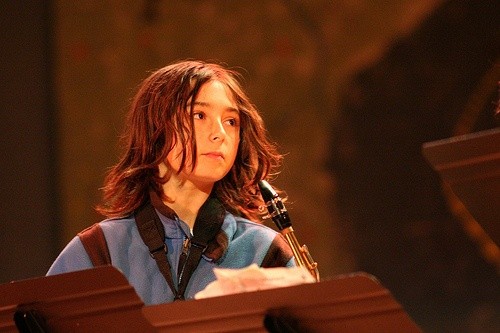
[46,59,310,307]
